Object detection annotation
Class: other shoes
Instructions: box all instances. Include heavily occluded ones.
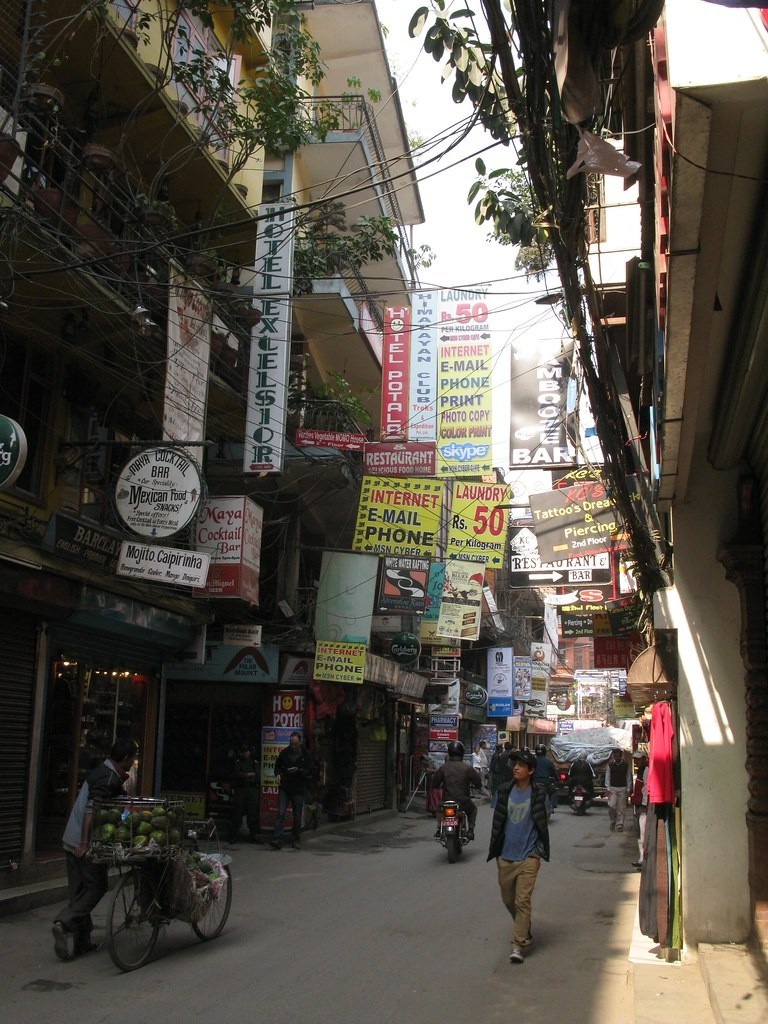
[610,823,615,830]
[251,836,258,843]
[632,861,642,867]
[521,935,532,946]
[617,824,623,831]
[74,941,96,955]
[434,830,440,837]
[292,841,301,849]
[270,839,282,849]
[510,947,523,962]
[465,831,474,840]
[52,921,69,960]
[229,835,240,844]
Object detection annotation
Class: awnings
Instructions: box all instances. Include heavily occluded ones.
[463,702,486,724]
[500,716,522,732]
[7,540,206,647]
[362,647,428,698]
[626,643,672,708]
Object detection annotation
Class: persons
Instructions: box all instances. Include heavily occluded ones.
[606,749,632,832]
[271,732,313,848]
[229,739,265,844]
[634,753,648,867]
[490,751,548,960]
[424,740,442,819]
[472,739,559,806]
[52,735,137,960]
[433,742,482,839]
[569,751,596,803]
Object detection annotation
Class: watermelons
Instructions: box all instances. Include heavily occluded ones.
[89,806,181,850]
[184,850,217,880]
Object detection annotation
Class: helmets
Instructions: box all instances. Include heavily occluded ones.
[535,744,546,755]
[448,741,465,755]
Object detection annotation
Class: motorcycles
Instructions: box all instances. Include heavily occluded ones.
[568,775,597,816]
[536,777,561,820]
[433,783,481,864]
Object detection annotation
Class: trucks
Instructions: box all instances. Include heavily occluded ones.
[546,745,634,797]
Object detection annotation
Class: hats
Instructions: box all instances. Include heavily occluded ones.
[632,750,647,759]
[240,745,251,752]
[509,751,537,768]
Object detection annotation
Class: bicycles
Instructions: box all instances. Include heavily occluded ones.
[95,817,236,970]
